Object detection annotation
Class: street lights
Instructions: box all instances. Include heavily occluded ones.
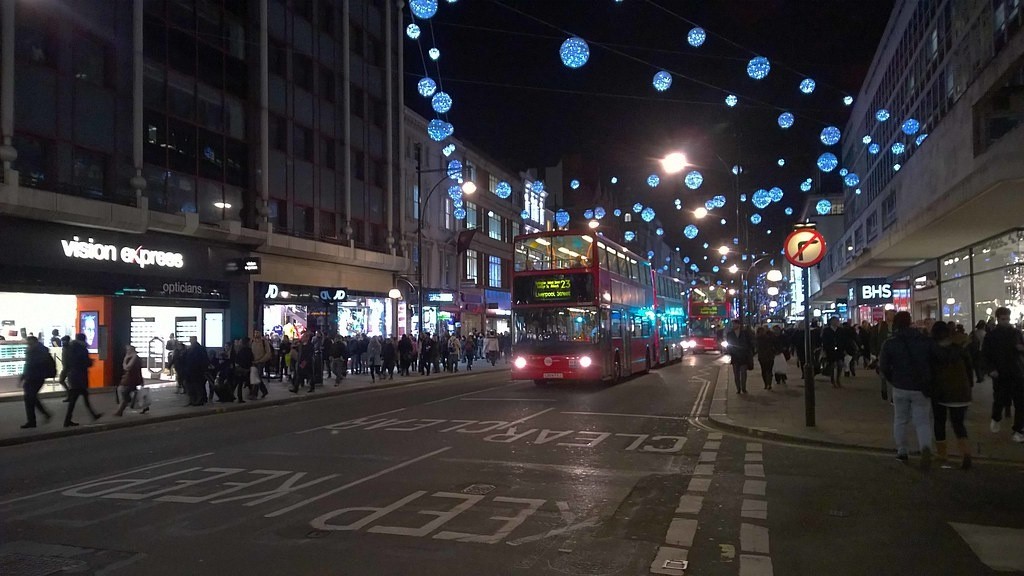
[655,140,748,329]
[415,162,477,335]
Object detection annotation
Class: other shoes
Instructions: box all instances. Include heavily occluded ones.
[307,387,315,392]
[896,452,910,462]
[737,387,741,394]
[63,420,80,427]
[289,389,298,393]
[742,389,747,393]
[112,410,123,417]
[990,419,1000,433]
[20,422,36,429]
[139,406,149,415]
[93,412,102,422]
[261,391,268,398]
[62,399,70,402]
[920,447,932,468]
[1012,432,1024,442]
[248,396,257,399]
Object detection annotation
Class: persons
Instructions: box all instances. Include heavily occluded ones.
[524,260,537,271]
[59,334,71,402]
[113,344,145,417]
[51,328,62,347]
[63,333,104,428]
[271,328,418,394]
[870,306,1024,473]
[165,329,281,408]
[573,255,585,267]
[417,328,513,376]
[727,317,888,395]
[17,336,57,430]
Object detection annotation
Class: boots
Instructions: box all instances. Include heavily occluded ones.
[959,439,972,469]
[238,396,246,403]
[936,441,948,462]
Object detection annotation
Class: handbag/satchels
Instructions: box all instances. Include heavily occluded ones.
[917,374,933,397]
[44,353,56,378]
[949,366,972,401]
[844,355,852,372]
[462,355,466,363]
[250,363,261,384]
[772,353,787,375]
[138,387,152,407]
[747,352,753,369]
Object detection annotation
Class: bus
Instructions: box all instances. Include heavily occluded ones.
[686,285,737,353]
[506,226,686,392]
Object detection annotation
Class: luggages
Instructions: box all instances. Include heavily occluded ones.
[204,373,235,403]
